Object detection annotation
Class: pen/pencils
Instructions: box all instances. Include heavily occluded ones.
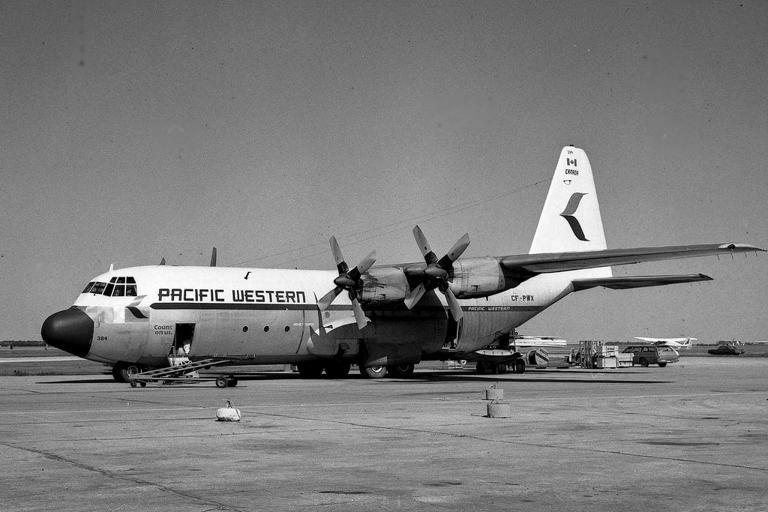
[621,344,679,367]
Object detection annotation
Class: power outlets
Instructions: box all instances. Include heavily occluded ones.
[708,345,745,355]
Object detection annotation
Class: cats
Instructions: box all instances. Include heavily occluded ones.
[633,337,698,350]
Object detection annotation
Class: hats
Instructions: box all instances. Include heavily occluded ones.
[40,145,768,388]
